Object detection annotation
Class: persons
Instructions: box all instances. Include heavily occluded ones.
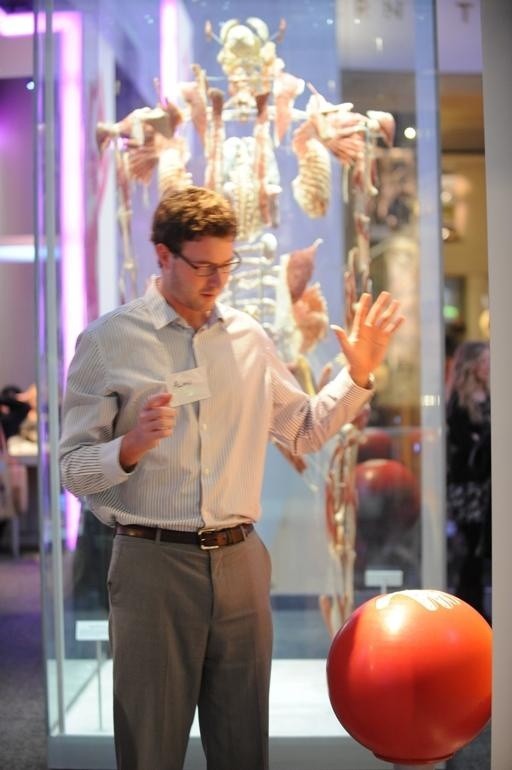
[57,185,405,769]
[446,342,489,619]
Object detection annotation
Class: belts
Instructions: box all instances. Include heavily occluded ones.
[115,521,254,550]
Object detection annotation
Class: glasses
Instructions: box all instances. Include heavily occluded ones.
[165,246,242,277]
